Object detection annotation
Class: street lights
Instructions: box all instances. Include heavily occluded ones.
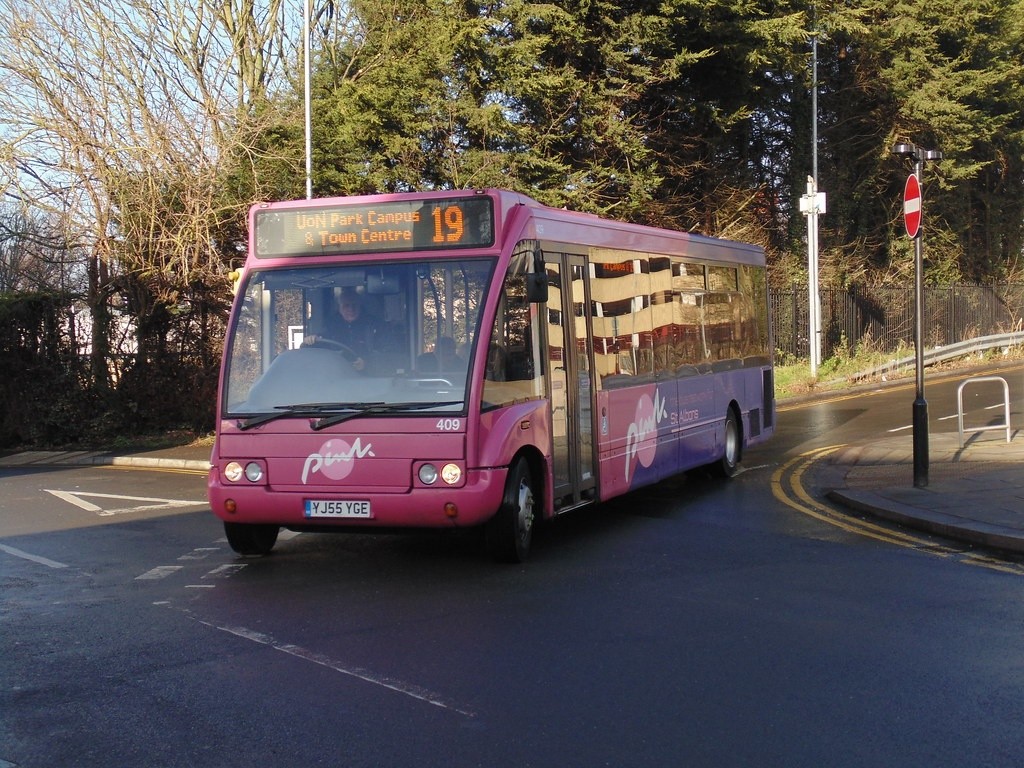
[891,143,943,489]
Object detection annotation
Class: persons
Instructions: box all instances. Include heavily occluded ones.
[416,337,464,398]
[303,289,404,371]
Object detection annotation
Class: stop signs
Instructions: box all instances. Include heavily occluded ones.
[903,173,922,239]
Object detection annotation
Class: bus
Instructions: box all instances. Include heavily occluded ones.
[206,185,779,565]
